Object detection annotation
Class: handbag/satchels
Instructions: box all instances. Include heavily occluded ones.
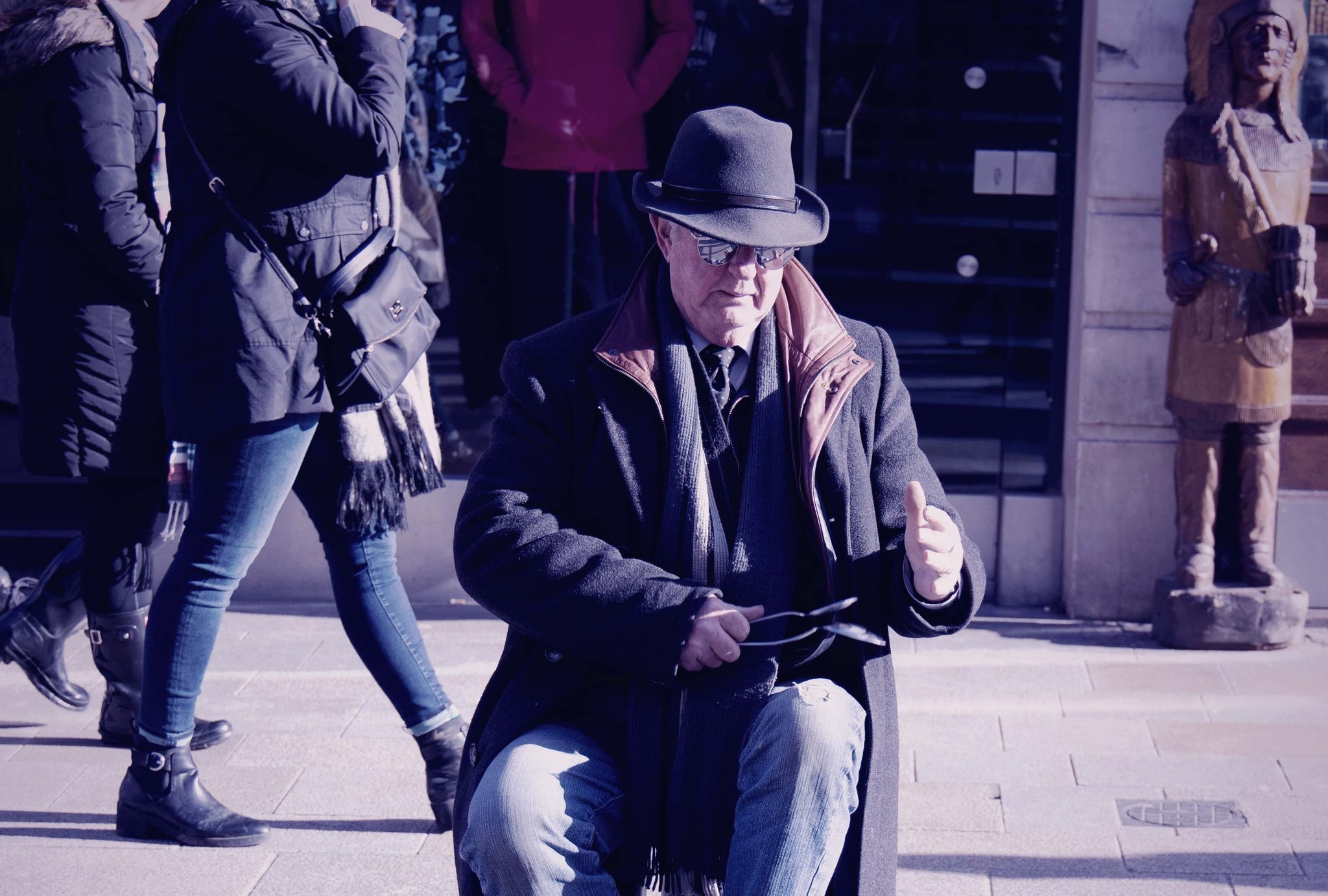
[318,226,441,405]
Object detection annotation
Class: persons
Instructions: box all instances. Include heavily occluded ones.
[109,0,485,851]
[452,2,701,182]
[0,0,236,760]
[431,94,989,896]
[373,0,477,198]
[1153,0,1312,596]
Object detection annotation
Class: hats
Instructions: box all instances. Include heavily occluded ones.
[633,106,831,248]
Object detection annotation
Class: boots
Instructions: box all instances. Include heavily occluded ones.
[0,534,93,712]
[86,604,232,750]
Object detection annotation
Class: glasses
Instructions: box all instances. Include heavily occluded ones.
[686,230,800,270]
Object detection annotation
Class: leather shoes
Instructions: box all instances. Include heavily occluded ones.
[410,703,470,833]
[116,734,271,848]
